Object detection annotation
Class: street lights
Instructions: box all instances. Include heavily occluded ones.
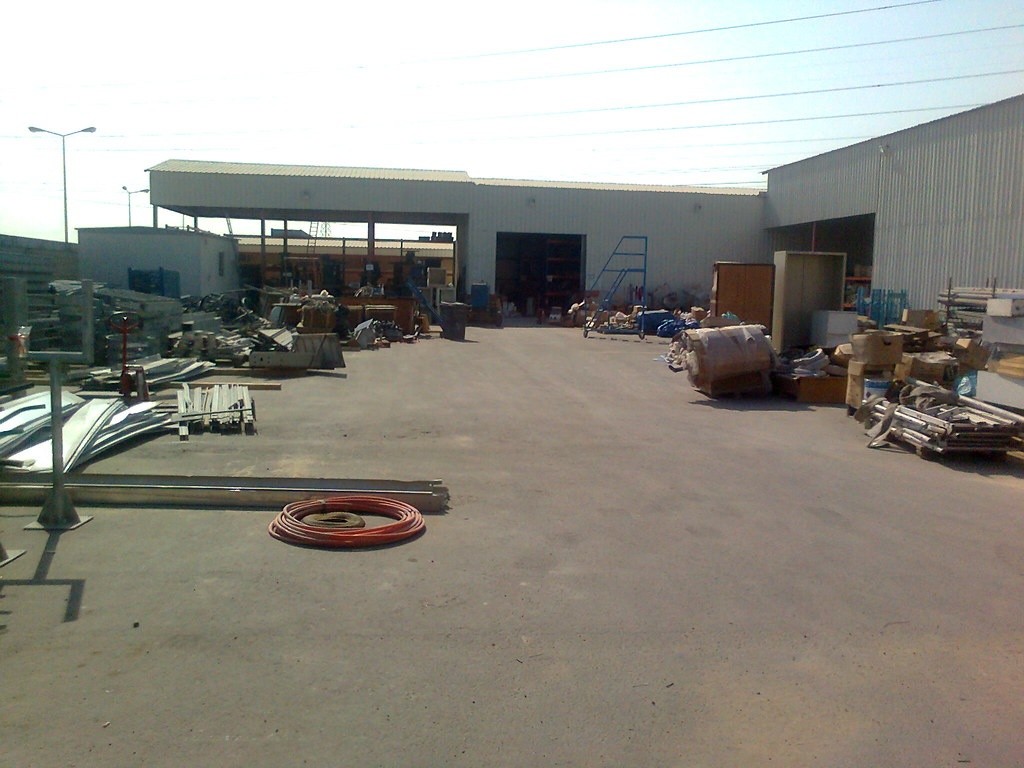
[29,126,96,242]
[122,186,150,227]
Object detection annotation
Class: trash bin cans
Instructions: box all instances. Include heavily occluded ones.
[437,301,470,342]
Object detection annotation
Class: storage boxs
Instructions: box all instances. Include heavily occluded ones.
[302,304,398,333]
[426,259,454,288]
[710,251,1024,411]
